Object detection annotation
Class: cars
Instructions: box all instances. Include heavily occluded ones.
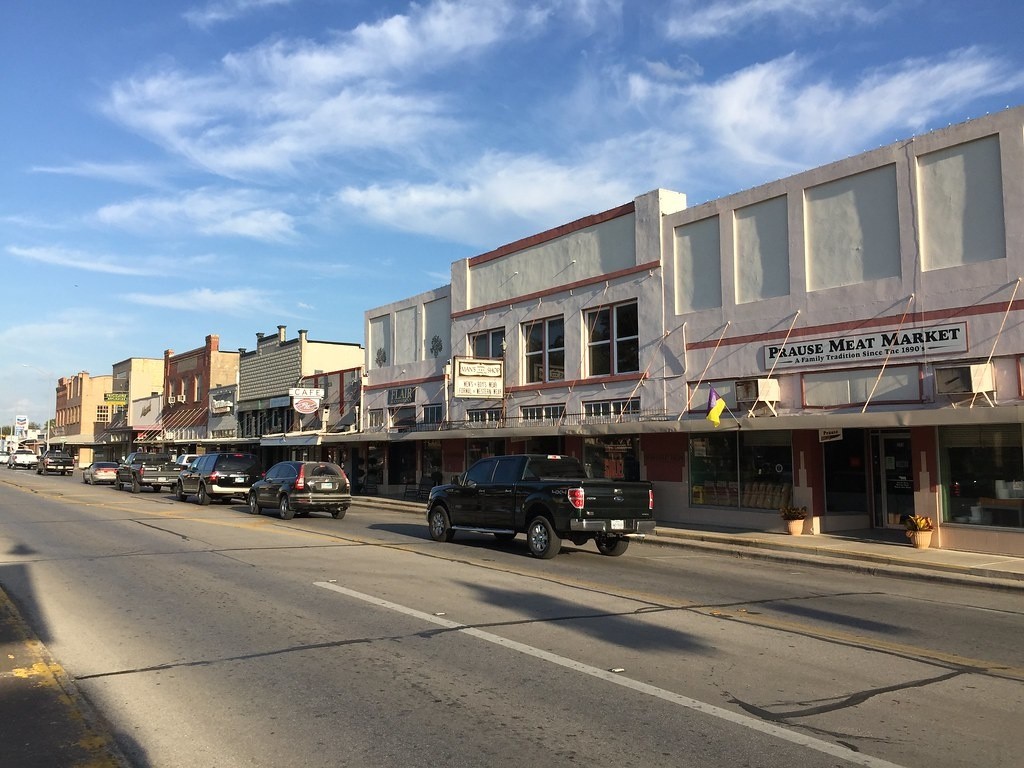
[83,461,119,485]
[0,451,12,464]
[8,449,40,470]
[247,461,352,521]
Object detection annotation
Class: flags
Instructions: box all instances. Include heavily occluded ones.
[704,387,726,427]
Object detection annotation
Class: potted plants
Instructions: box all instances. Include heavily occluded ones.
[780,505,807,536]
[431,471,442,486]
[904,514,935,549]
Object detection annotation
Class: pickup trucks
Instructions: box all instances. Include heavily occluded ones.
[426,454,657,560]
[36,448,75,477]
[115,451,188,495]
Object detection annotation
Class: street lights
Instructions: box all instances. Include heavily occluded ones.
[22,363,52,451]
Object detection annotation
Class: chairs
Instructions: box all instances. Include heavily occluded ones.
[404,477,436,502]
[354,474,378,495]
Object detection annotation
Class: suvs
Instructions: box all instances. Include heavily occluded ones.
[175,451,266,507]
[174,453,202,469]
